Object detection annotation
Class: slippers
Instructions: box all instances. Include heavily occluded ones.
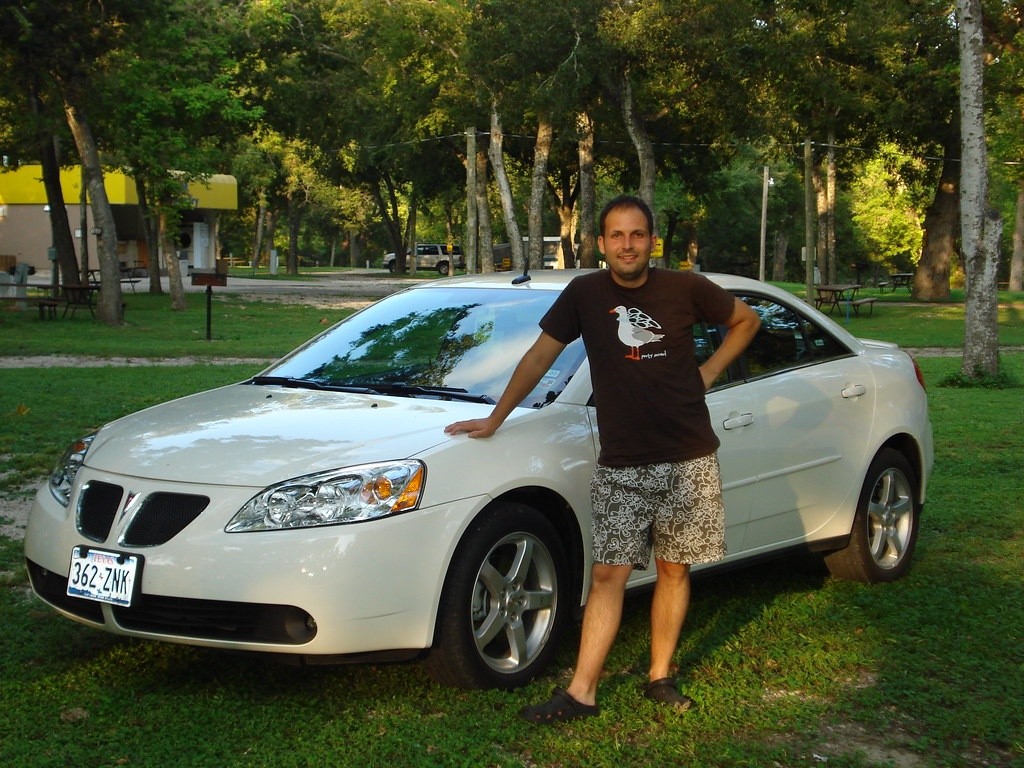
[518,687,600,724]
[645,677,696,713]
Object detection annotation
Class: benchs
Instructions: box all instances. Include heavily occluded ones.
[879,282,888,294]
[0,261,147,323]
[802,297,825,308]
[850,298,877,317]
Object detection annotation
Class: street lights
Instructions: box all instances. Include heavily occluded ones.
[759,166,774,283]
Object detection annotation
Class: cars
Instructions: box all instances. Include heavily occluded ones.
[25,268,934,694]
[382,243,466,276]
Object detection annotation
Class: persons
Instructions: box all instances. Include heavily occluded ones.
[443,195,760,724]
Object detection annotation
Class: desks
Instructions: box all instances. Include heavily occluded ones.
[78,268,101,281]
[890,273,914,293]
[816,284,863,316]
[58,284,100,317]
[0,282,58,320]
